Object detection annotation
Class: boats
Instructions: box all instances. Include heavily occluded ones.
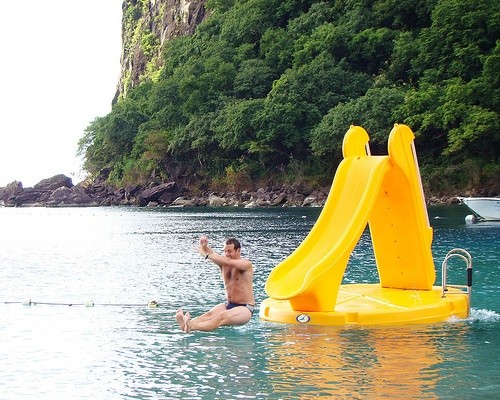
[457,197,500,222]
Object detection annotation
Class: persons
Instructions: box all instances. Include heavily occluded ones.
[174,234,255,333]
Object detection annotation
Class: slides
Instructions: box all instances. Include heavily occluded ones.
[264,156,388,300]
